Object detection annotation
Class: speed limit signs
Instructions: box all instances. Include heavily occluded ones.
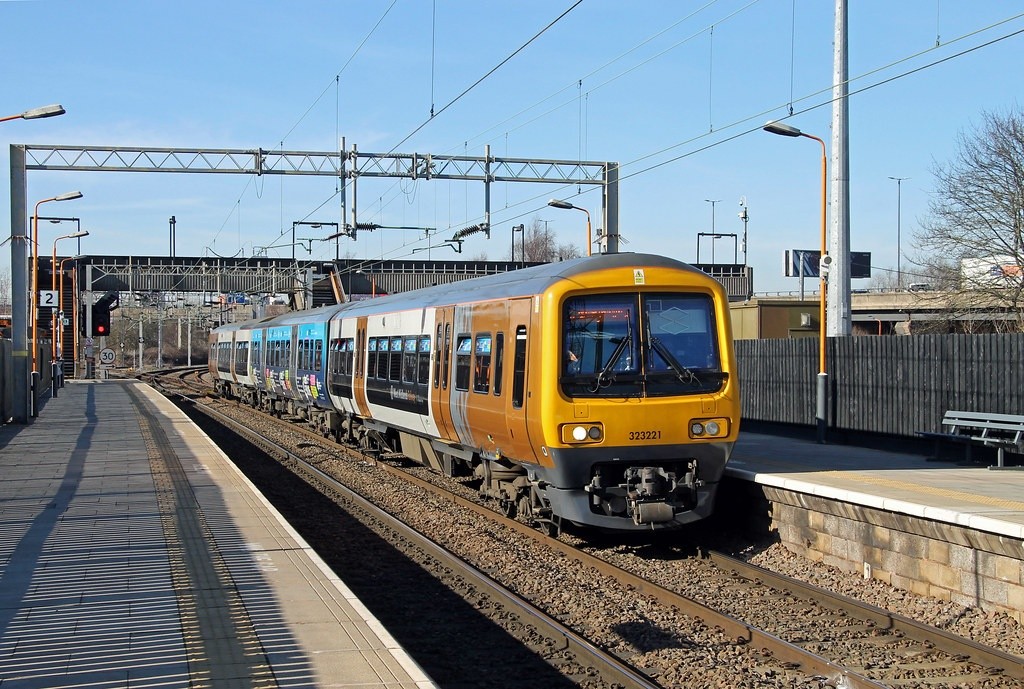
[100,348,116,364]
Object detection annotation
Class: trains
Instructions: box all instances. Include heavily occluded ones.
[209,254,740,537]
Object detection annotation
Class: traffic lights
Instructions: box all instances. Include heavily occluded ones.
[93,306,110,336]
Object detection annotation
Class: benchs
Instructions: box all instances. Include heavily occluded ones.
[914,411,1024,470]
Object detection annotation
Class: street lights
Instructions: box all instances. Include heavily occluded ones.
[60,254,89,388]
[548,200,592,255]
[764,121,831,444]
[888,176,912,288]
[30,191,83,418]
[704,197,723,263]
[53,230,90,398]
